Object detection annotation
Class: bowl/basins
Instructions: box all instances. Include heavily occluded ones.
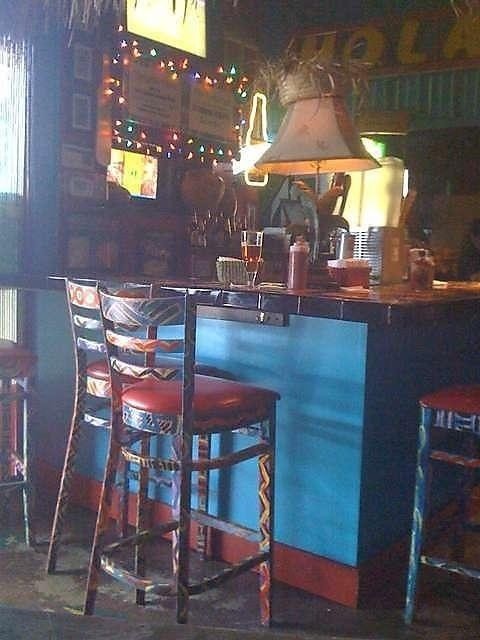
[330,266,372,287]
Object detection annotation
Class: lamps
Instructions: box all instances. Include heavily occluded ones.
[254,94,384,290]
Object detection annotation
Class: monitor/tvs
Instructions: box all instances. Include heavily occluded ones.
[110,146,160,200]
[124,0,208,60]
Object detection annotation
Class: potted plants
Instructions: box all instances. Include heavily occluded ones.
[246,46,373,105]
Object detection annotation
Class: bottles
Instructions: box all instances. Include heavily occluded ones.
[407,250,436,291]
[286,236,310,291]
[245,95,270,185]
[186,207,250,248]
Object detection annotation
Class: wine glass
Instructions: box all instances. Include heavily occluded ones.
[240,230,264,289]
